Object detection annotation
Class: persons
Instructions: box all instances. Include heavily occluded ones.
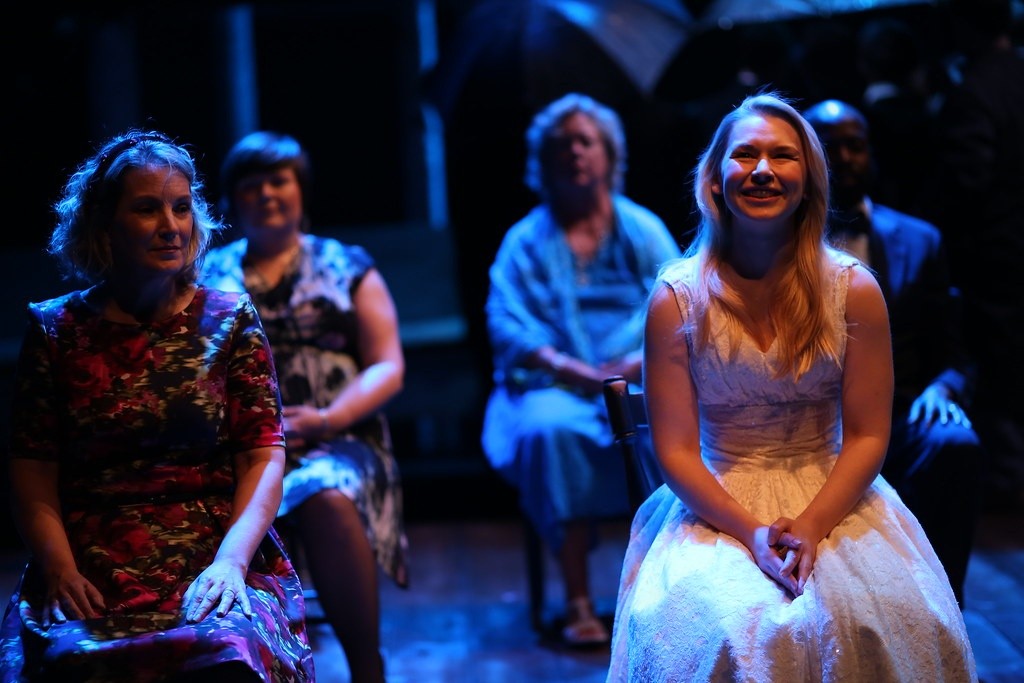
[604,93,978,683]
[0,132,315,683]
[479,94,682,648]
[802,98,976,612]
[198,133,406,683]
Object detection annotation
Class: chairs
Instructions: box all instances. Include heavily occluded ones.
[523,375,654,632]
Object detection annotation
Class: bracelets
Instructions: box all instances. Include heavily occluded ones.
[321,408,328,430]
